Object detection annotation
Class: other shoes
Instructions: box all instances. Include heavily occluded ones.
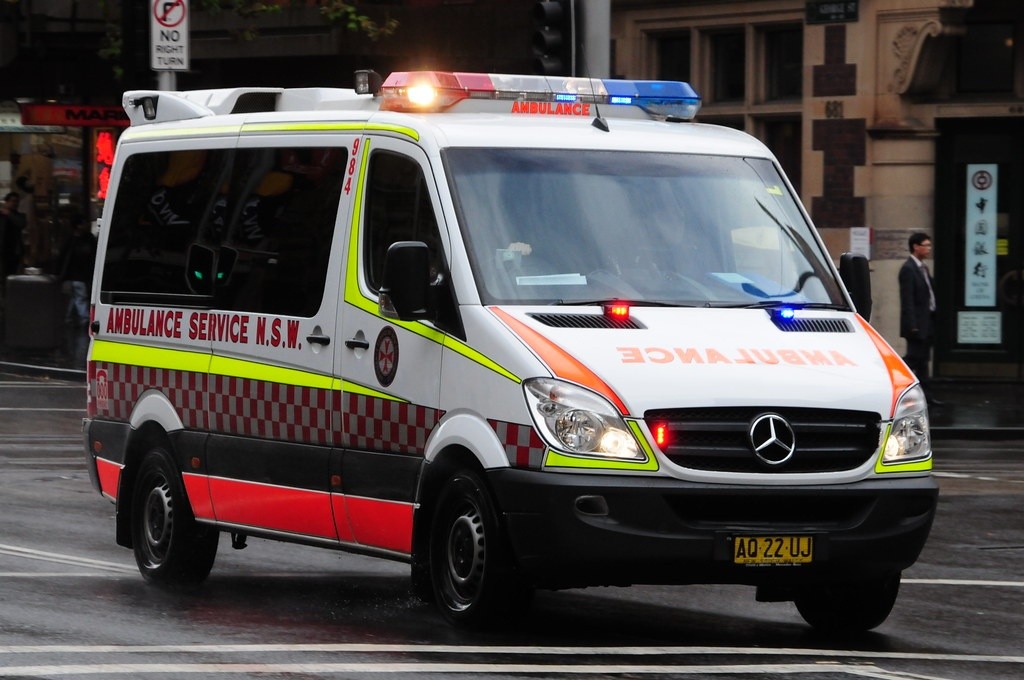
[927,397,945,406]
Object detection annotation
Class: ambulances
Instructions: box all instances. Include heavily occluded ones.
[81,65,943,641]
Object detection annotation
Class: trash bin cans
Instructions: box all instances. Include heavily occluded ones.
[5,273,61,362]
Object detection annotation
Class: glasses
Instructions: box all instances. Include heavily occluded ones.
[920,244,932,248]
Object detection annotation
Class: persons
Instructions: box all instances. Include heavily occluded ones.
[504,241,534,257]
[0,192,97,383]
[899,231,939,380]
